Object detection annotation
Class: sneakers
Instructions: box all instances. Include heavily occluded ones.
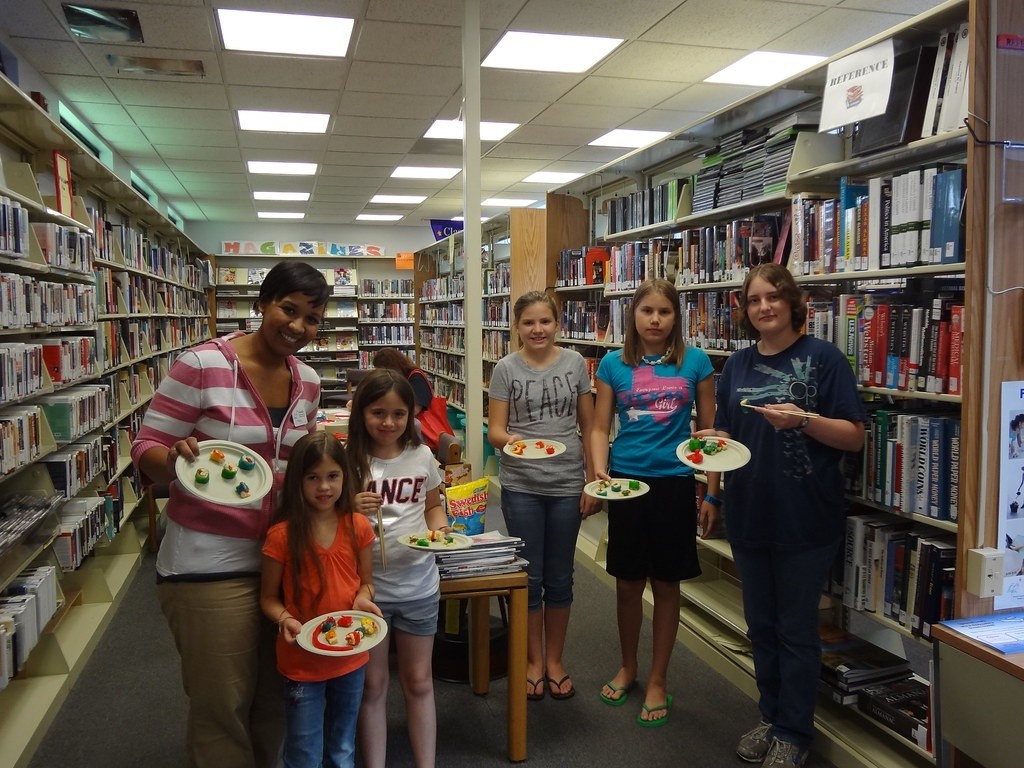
[760,735,811,768]
[736,719,775,763]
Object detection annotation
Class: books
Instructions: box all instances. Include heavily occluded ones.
[0,193,529,688]
[550,21,969,752]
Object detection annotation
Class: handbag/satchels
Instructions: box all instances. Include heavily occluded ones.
[406,368,456,450]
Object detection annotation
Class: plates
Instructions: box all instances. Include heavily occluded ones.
[174,440,274,506]
[397,531,473,552]
[583,478,650,499]
[295,609,390,655]
[503,438,566,459]
[675,435,753,472]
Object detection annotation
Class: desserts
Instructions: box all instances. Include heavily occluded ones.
[321,615,376,646]
[511,440,554,455]
[687,435,728,464]
[596,478,639,497]
[408,529,456,547]
[196,449,256,498]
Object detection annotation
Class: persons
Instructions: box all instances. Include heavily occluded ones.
[690,263,864,768]
[590,280,722,728]
[487,291,603,701]
[129,259,329,768]
[260,431,383,768]
[343,369,453,768]
[347,348,455,459]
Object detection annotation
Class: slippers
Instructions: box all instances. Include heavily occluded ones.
[600,677,636,706]
[637,694,672,727]
[544,669,576,699]
[525,677,545,700]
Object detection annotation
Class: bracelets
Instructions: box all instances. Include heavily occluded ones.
[794,409,810,430]
[438,526,449,530]
[704,495,722,512]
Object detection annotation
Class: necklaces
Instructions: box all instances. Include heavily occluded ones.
[641,346,673,365]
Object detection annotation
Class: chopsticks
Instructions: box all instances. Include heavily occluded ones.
[378,505,387,571]
[741,403,820,420]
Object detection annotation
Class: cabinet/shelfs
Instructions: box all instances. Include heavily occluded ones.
[361,0,1024,768]
[215,284,359,399]
[0,68,215,768]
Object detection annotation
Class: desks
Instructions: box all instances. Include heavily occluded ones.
[438,569,531,762]
